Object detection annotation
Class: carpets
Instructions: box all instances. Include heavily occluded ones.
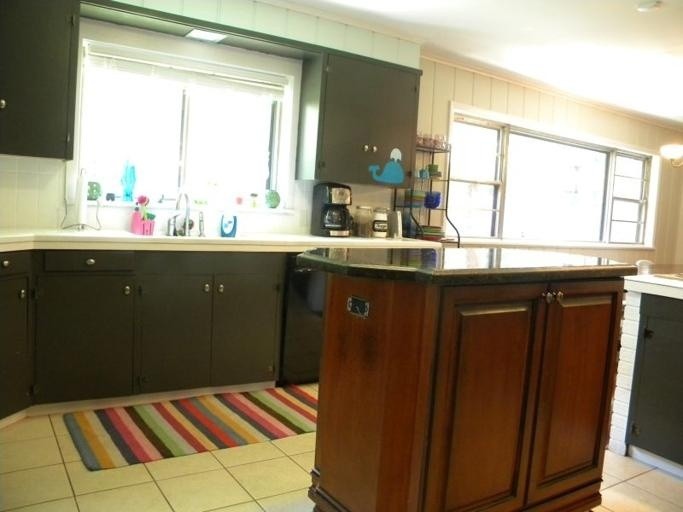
[63,382,319,471]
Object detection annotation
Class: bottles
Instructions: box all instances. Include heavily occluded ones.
[353,204,390,238]
[416,130,448,150]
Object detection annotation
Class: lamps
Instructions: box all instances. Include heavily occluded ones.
[659,143,683,168]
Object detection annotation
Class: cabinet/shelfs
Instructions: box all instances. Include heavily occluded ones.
[295,52,423,190]
[0,0,80,161]
[391,143,460,249]
[307,272,624,512]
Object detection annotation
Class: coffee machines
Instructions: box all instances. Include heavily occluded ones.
[309,181,353,237]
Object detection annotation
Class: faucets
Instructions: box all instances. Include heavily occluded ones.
[174,191,191,236]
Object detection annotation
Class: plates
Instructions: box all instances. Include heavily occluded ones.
[416,224,447,241]
[404,190,441,208]
